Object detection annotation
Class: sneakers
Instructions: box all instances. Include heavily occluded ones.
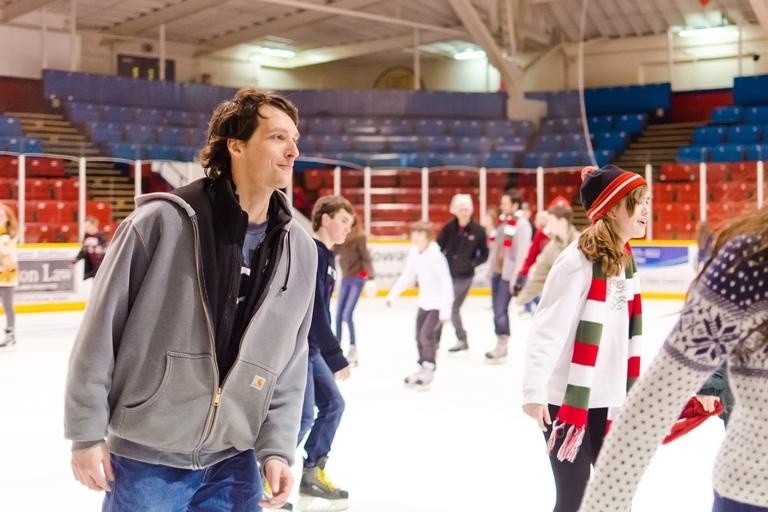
[448,342,467,352]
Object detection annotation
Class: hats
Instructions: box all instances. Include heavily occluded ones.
[580,165,647,223]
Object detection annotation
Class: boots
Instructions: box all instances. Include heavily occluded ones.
[300,456,348,499]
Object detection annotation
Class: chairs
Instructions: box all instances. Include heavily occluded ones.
[0,67,767,254]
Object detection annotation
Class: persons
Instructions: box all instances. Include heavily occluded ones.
[63,87,319,512]
[1,204,19,347]
[332,214,376,364]
[487,189,585,361]
[69,215,109,304]
[520,163,649,512]
[435,192,490,353]
[695,355,734,430]
[258,194,357,511]
[573,204,768,512]
[383,219,454,383]
